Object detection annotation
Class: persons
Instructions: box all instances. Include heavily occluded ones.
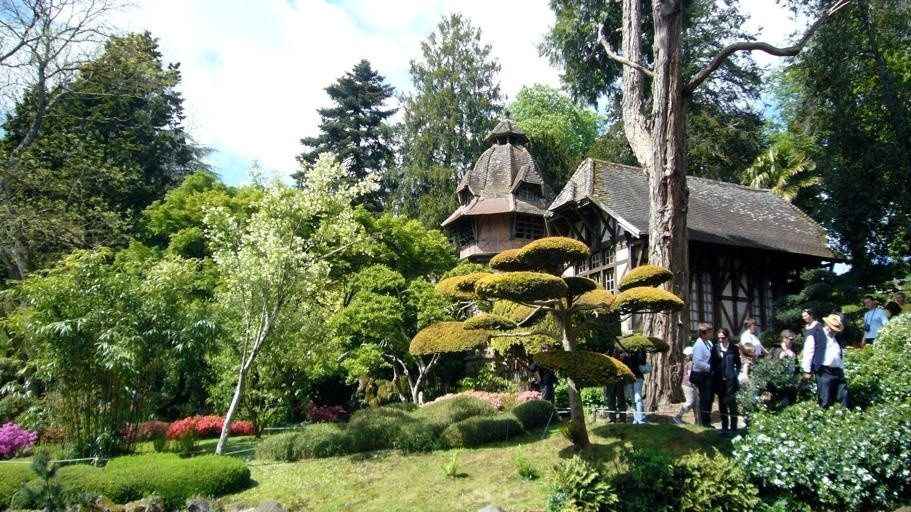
[623,348,648,424]
[602,349,628,425]
[530,344,563,422]
[673,290,903,430]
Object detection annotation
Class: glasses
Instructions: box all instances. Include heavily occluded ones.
[716,335,727,340]
[785,336,795,342]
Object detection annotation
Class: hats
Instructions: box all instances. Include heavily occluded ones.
[821,314,845,333]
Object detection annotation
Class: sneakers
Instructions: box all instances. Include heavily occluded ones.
[672,414,682,425]
